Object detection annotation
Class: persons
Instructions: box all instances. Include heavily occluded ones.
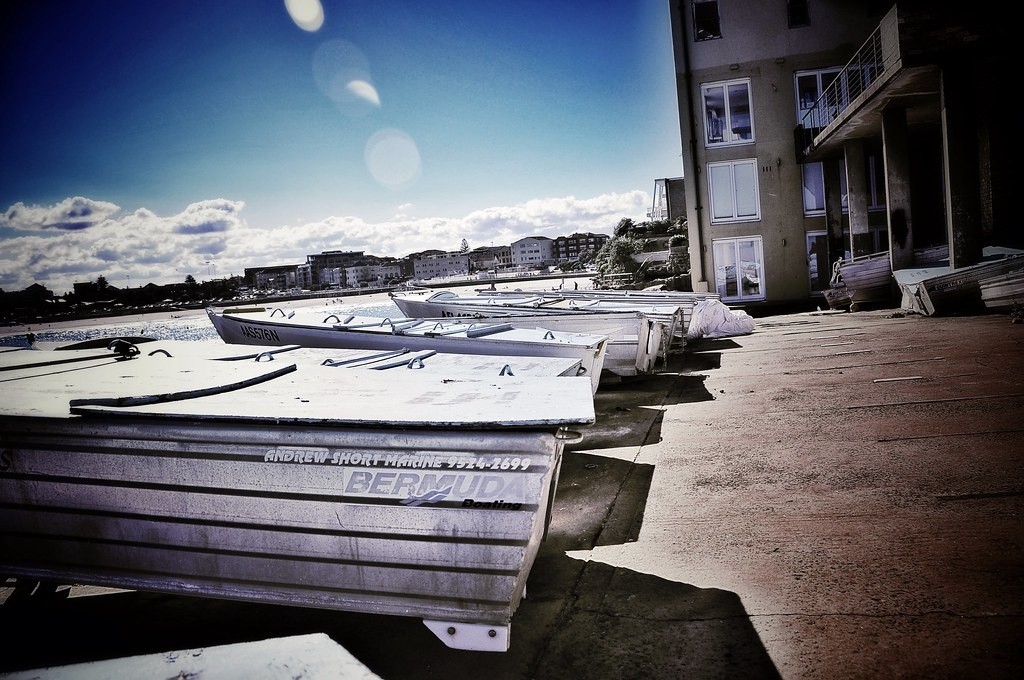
[21,326,39,350]
[495,268,497,276]
[470,263,479,275]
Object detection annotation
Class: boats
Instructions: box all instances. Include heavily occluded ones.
[821,244,1024,325]
[0,287,723,653]
